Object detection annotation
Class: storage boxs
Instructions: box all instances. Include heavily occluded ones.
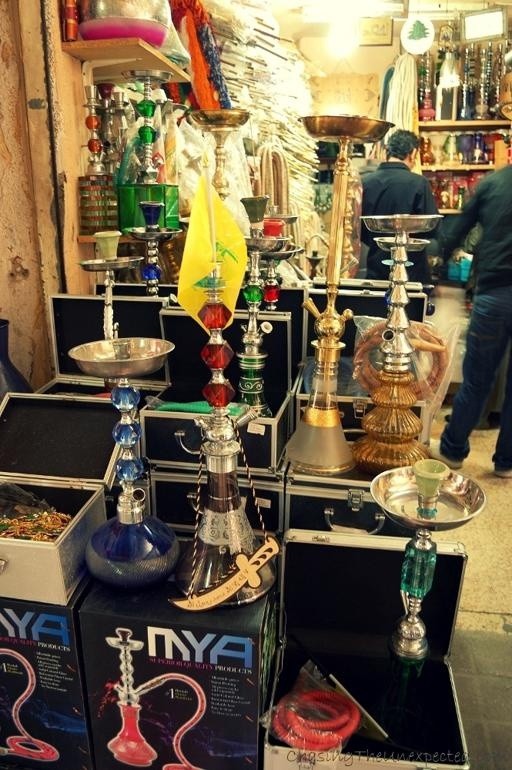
[1,391,137,607]
[76,537,281,770]
[0,573,102,768]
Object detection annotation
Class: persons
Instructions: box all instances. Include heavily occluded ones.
[360,129,441,291]
[428,164,512,479]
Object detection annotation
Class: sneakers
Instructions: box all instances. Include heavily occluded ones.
[428,439,463,469]
[495,470,512,478]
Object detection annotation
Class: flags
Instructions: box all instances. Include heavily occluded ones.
[177,175,247,338]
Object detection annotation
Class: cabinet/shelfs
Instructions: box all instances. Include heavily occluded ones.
[61,36,187,245]
[414,120,512,215]
